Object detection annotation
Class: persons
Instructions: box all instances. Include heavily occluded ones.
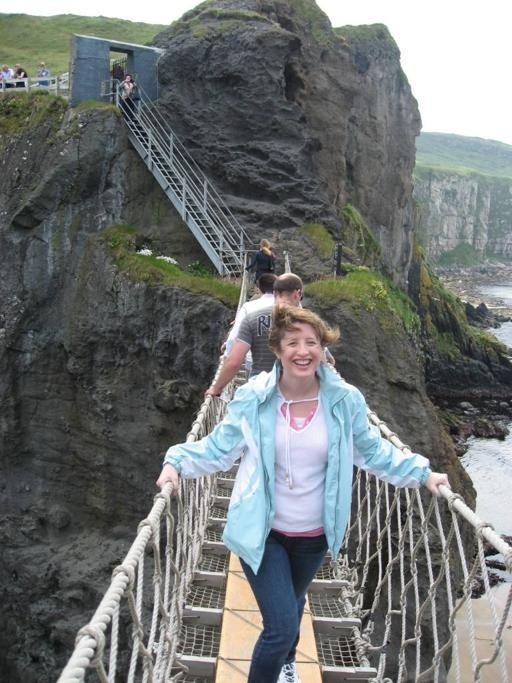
[121,75,136,116]
[221,273,335,382]
[154,308,451,683]
[204,273,304,396]
[38,61,49,86]
[0,62,28,88]
[245,238,276,282]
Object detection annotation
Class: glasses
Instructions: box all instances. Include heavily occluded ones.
[296,288,303,300]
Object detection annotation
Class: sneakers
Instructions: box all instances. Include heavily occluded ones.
[278,659,299,682]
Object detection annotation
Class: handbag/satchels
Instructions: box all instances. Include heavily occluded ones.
[130,85,142,101]
